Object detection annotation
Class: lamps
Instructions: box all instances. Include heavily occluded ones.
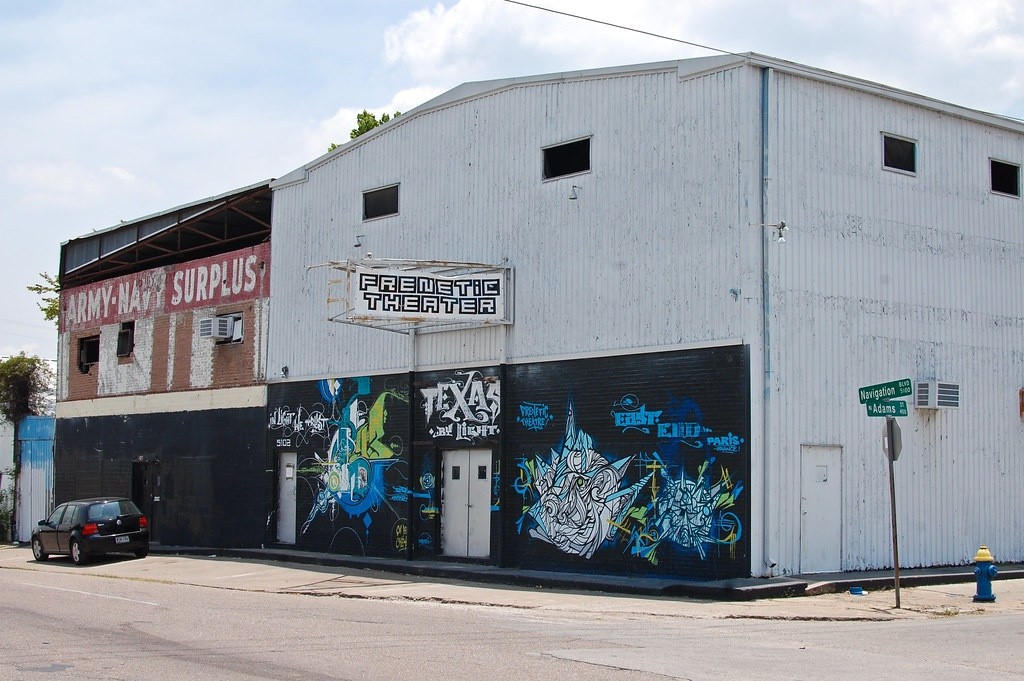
[568,185,582,200]
[352,235,365,248]
[748,221,789,244]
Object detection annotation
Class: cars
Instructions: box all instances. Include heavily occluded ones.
[30,496,149,564]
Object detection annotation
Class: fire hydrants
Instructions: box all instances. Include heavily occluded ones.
[972,545,998,602]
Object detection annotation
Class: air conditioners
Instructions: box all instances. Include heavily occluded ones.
[914,381,960,411]
[198,316,234,338]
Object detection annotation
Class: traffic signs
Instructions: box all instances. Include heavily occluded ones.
[859,377,913,417]
